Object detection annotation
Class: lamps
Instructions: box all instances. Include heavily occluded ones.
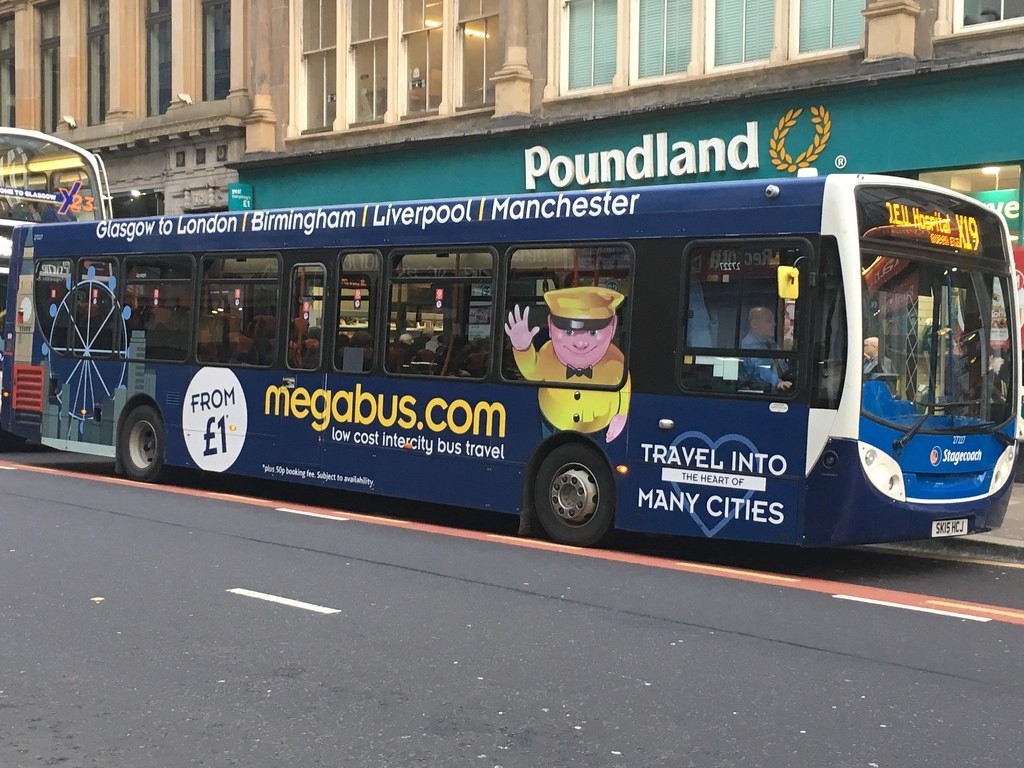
[426,17,491,39]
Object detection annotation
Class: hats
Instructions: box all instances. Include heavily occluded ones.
[399,334,414,345]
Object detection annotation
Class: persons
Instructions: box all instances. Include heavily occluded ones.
[0,308,7,338]
[739,305,793,394]
[917,318,933,384]
[863,336,898,395]
[37,283,522,378]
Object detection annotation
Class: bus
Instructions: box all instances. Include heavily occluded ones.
[1,170,1024,547]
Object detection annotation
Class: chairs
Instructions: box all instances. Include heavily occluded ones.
[77,301,406,372]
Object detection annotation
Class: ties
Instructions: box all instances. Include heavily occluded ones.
[767,342,783,378]
